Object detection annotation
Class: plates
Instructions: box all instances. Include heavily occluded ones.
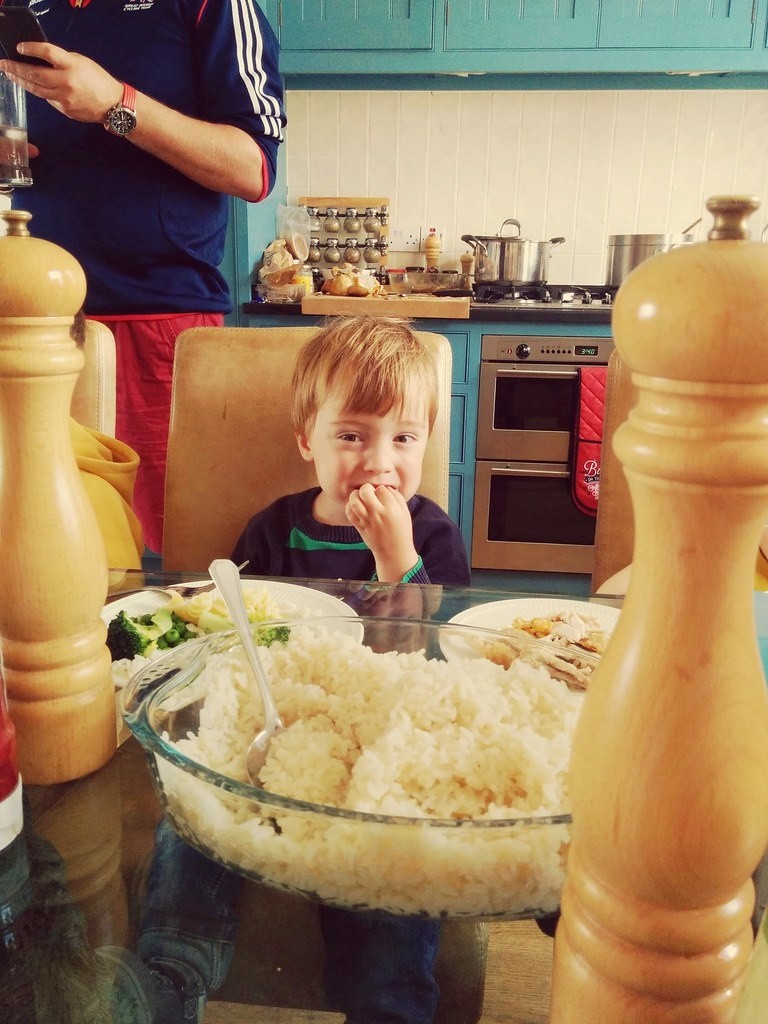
[103,576,363,688]
[438,598,623,701]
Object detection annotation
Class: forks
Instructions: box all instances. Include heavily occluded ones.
[104,559,251,601]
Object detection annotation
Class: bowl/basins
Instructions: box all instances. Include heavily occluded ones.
[120,613,601,924]
[404,272,457,291]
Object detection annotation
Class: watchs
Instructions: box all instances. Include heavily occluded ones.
[103,81,138,139]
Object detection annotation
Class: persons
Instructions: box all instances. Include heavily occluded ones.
[98,318,470,1024]
[0,0,287,554]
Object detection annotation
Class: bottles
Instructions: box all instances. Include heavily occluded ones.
[292,259,325,297]
[387,228,474,292]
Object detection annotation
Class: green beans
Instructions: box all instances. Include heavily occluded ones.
[131,611,197,649]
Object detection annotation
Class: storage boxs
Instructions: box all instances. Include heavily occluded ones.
[257,283,305,303]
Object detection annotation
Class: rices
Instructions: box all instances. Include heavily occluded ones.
[154,626,579,915]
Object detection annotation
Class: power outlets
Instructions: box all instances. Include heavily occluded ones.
[388,226,420,253]
[420,227,445,254]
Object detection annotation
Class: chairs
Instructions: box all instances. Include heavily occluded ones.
[158,325,453,572]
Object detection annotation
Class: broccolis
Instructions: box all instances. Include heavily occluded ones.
[245,614,289,648]
[106,610,174,661]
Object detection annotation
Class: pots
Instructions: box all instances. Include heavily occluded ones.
[460,218,566,285]
[605,233,693,287]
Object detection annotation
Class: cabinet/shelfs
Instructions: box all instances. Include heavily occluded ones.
[272,0,438,75]
[216,0,287,312]
[239,309,479,574]
[437,0,602,74]
[597,0,762,72]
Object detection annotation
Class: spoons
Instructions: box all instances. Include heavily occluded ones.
[206,557,285,791]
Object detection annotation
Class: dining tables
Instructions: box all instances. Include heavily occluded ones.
[0,566,768,1024]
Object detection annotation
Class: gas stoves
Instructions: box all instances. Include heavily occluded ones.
[468,285,621,322]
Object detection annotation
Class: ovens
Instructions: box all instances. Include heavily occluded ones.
[472,334,614,573]
